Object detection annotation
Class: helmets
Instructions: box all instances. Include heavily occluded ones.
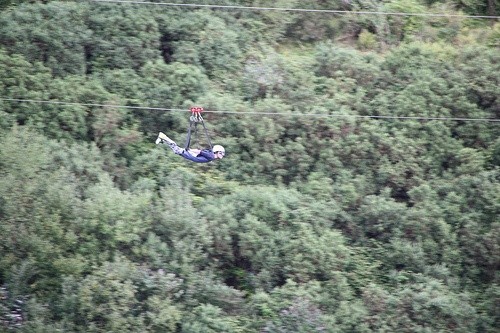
[213,145,225,152]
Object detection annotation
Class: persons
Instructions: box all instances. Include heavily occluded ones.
[155,132,225,163]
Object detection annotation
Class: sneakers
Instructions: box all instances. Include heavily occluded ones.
[156,131,165,144]
